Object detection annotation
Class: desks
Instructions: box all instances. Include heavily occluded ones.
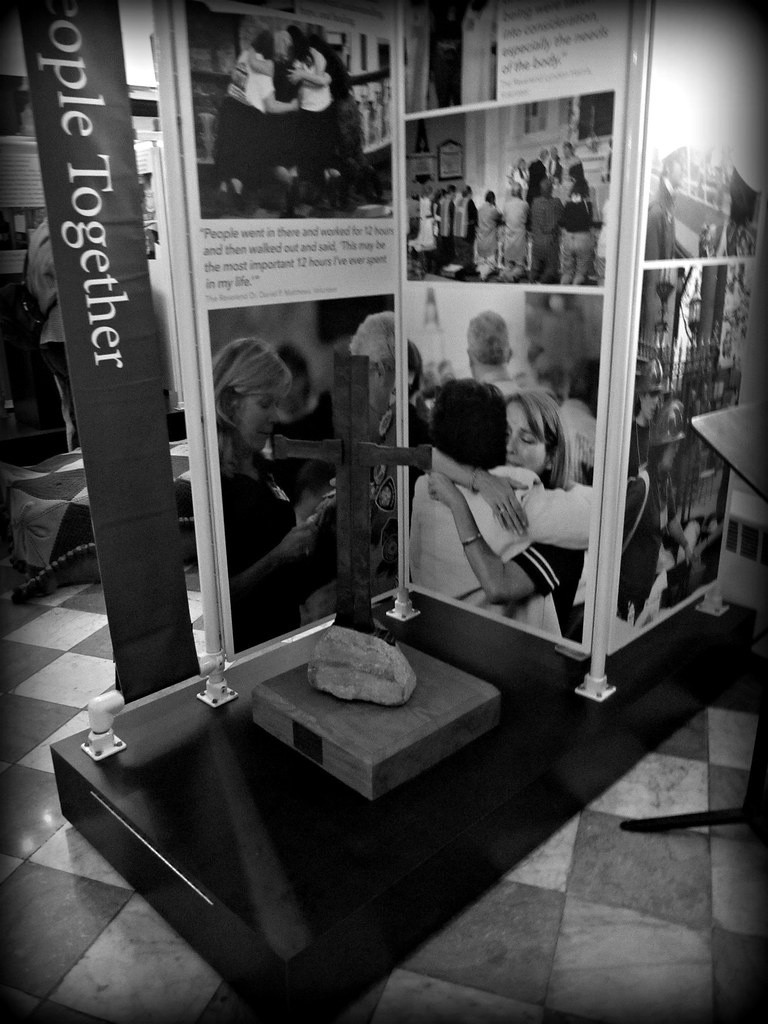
[49,596,756,1023]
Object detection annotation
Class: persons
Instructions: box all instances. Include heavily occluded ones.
[2,1,766,658]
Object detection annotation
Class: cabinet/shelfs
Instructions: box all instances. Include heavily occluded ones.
[384,1,768,707]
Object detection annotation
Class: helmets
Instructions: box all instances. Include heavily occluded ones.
[637,356,664,392]
[649,399,686,445]
